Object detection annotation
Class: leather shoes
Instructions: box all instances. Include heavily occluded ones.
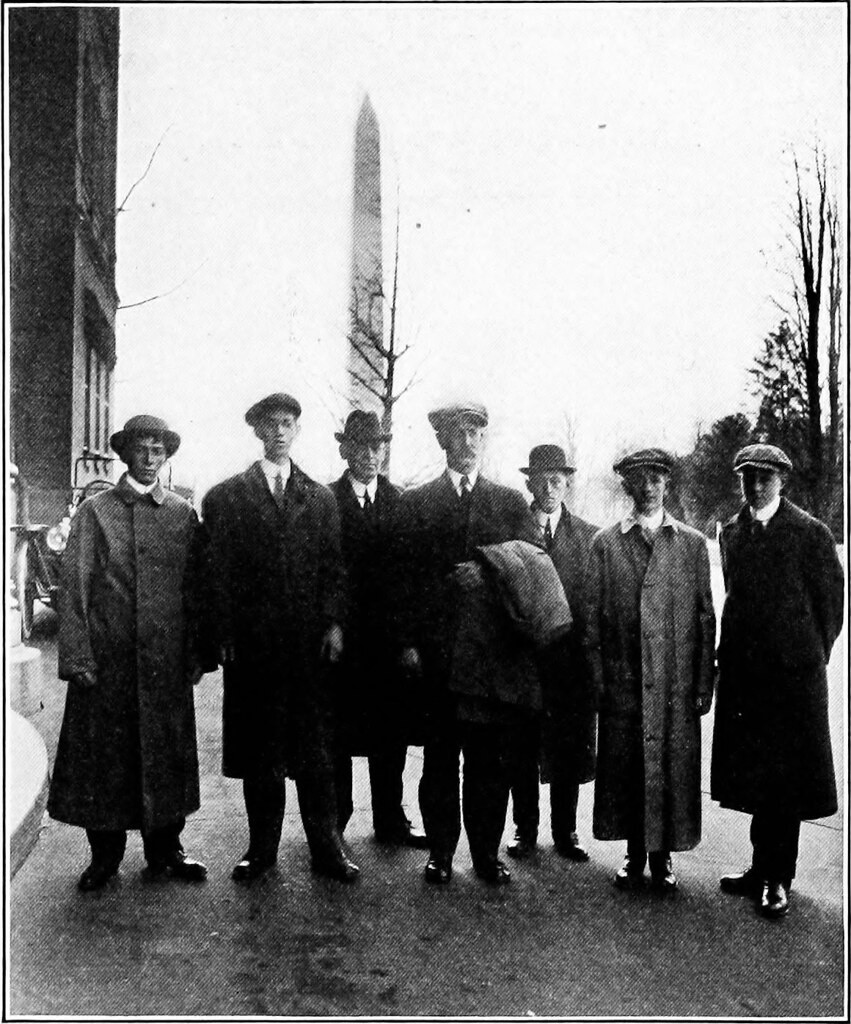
[232,848,275,882]
[479,860,512,887]
[761,876,787,917]
[506,835,534,858]
[616,853,645,890]
[78,853,121,892]
[149,853,206,882]
[314,851,361,881]
[561,844,589,862]
[719,874,766,895]
[375,821,429,849]
[424,855,450,885]
[651,860,677,894]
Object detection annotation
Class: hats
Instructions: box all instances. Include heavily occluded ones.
[244,392,301,429]
[428,403,488,433]
[334,409,392,444]
[520,445,577,475]
[612,448,674,474]
[110,415,180,458]
[734,445,793,480]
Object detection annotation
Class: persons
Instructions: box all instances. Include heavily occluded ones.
[388,400,535,885]
[327,410,425,847]
[577,448,715,893]
[46,415,208,888]
[192,392,361,882]
[508,444,598,864]
[710,443,845,919]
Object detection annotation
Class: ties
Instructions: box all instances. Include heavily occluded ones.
[754,519,763,538]
[645,526,653,543]
[460,475,470,504]
[363,492,373,521]
[274,473,285,511]
[544,519,553,550]
